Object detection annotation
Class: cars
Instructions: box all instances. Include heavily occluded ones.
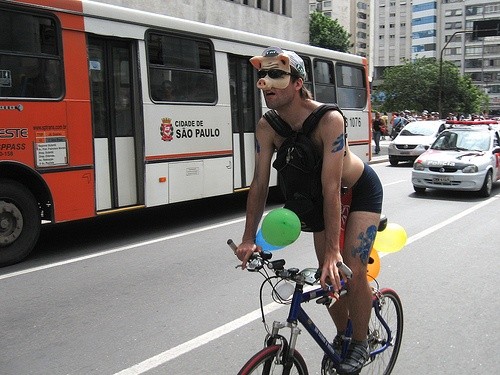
[387,117,448,167]
[410,120,500,197]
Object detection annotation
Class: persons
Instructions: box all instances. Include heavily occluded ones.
[447,113,489,121]
[383,110,439,137]
[235,49,382,375]
[162,80,176,99]
[372,112,385,154]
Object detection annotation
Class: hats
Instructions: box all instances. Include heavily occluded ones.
[260,46,307,80]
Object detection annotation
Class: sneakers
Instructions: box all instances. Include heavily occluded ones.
[336,341,371,375]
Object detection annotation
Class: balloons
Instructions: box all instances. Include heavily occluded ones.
[255,229,286,250]
[261,208,301,246]
[375,223,407,252]
[364,248,380,281]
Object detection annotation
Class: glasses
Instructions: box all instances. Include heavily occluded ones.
[257,69,299,79]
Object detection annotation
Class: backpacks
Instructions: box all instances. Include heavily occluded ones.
[262,104,347,232]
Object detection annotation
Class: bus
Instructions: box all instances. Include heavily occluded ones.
[0,0,373,269]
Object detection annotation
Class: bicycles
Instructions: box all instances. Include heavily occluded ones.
[226,218,405,374]
